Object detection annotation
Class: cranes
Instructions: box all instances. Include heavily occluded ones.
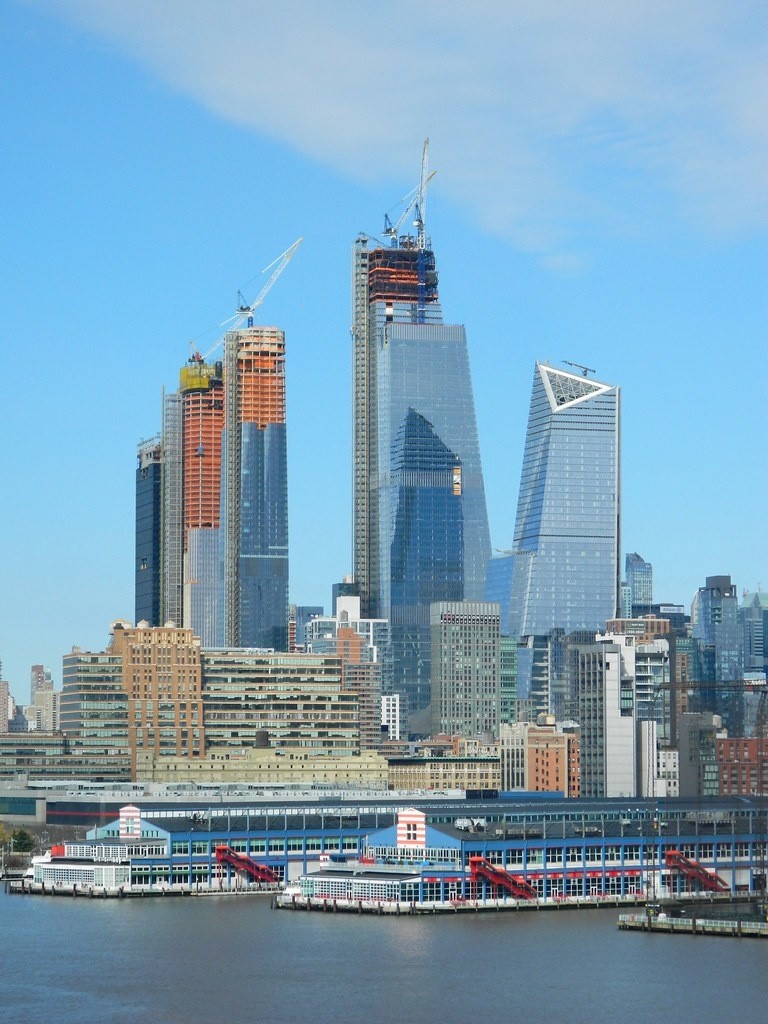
[355,137,439,325]
[181,236,303,367]
